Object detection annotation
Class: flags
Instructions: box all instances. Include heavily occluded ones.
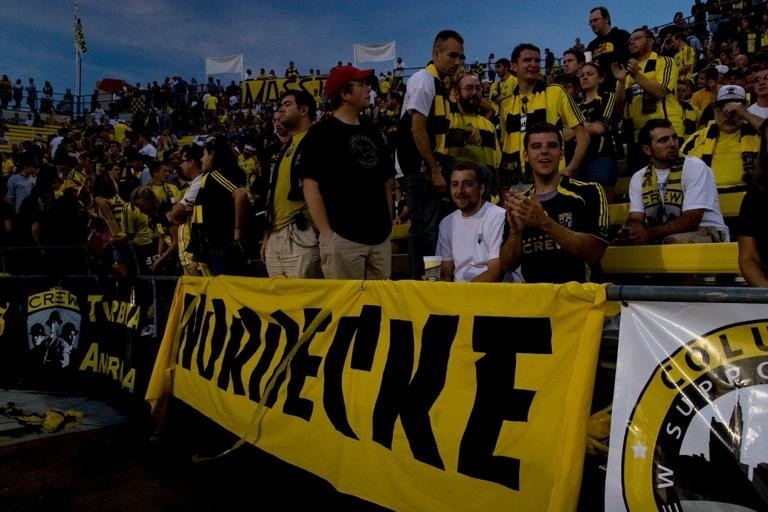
[72,1,87,57]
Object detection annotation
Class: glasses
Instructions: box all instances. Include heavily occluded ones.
[627,33,649,42]
[753,74,768,83]
[458,86,484,93]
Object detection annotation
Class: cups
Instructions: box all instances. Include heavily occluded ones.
[423,256,442,281]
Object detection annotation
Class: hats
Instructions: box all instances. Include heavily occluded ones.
[325,64,375,97]
[711,84,748,106]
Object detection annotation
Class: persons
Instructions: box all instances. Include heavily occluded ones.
[28,309,77,371]
[586,6,638,160]
[567,75,582,106]
[544,48,555,74]
[681,84,764,284]
[611,28,686,175]
[346,61,354,68]
[225,79,271,225]
[362,56,413,226]
[427,73,503,257]
[675,0,768,132]
[184,139,248,276]
[306,66,336,125]
[1,73,179,338]
[496,43,592,207]
[564,48,587,83]
[180,74,230,146]
[574,37,586,52]
[472,50,496,102]
[489,58,518,117]
[611,116,731,285]
[299,65,397,279]
[434,161,522,282]
[641,12,694,78]
[499,121,611,283]
[563,60,618,189]
[736,118,768,288]
[169,143,203,277]
[338,59,343,68]
[394,30,467,280]
[256,59,300,161]
[259,88,322,278]
